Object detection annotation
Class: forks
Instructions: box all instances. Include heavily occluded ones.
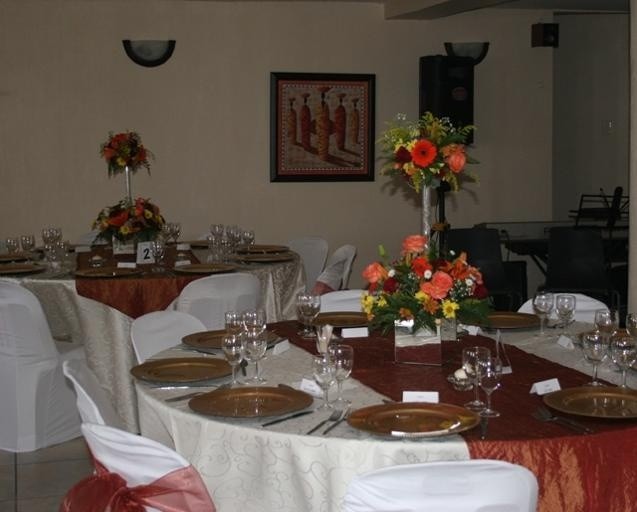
[306,410,343,435]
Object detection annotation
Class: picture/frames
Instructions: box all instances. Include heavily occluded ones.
[270,72,376,181]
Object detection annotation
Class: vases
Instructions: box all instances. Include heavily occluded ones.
[112,235,136,255]
[394,316,457,366]
[422,185,432,246]
[124,166,130,196]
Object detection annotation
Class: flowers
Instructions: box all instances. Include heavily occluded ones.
[90,195,166,243]
[376,112,482,194]
[360,235,497,335]
[98,128,156,180]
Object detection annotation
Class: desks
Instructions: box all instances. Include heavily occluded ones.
[480,221,630,256]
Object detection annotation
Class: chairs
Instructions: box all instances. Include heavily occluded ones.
[517,292,609,323]
[327,244,357,289]
[312,256,348,296]
[343,459,539,512]
[130,310,207,364]
[63,359,124,427]
[447,228,523,312]
[177,273,261,331]
[0,279,87,509]
[320,289,369,312]
[62,421,217,511]
[292,237,328,292]
[544,226,621,309]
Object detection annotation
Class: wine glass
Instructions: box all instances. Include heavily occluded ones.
[222,307,268,387]
[581,309,635,388]
[308,343,354,416]
[533,294,577,338]
[295,292,325,341]
[5,223,255,276]
[460,346,503,419]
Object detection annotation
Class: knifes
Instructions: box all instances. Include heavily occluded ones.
[188,383,314,419]
[323,407,353,434]
[165,391,203,403]
[261,410,315,428]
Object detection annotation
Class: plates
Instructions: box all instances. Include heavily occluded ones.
[130,357,233,384]
[1,239,295,278]
[471,311,542,330]
[181,327,280,351]
[345,402,481,440]
[581,325,637,348]
[542,389,636,419]
[309,313,379,327]
